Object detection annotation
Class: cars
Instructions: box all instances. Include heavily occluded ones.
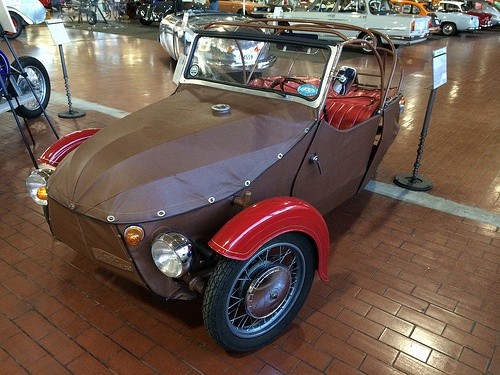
[25,17,407,351]
[133,0,500,36]
[159,9,277,80]
[0,0,46,39]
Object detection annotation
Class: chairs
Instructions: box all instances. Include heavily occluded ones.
[321,83,380,130]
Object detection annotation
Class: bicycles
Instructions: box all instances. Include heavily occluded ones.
[39,0,133,24]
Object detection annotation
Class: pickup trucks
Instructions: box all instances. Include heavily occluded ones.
[274,0,429,54]
